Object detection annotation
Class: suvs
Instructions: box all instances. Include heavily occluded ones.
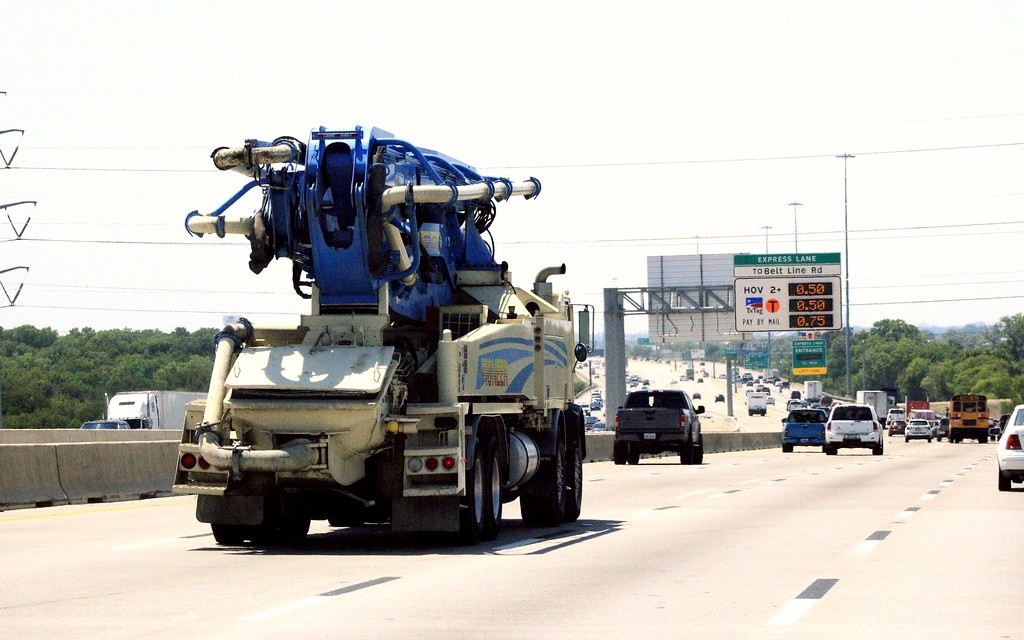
[904,419,931,444]
[791,390,800,399]
[886,408,905,424]
[931,418,949,442]
[824,403,885,455]
[782,409,829,452]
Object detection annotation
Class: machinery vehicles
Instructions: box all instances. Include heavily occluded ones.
[171,126,594,549]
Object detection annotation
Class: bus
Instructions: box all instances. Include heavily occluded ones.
[946,392,989,444]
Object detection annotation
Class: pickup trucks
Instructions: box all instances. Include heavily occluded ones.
[612,391,707,465]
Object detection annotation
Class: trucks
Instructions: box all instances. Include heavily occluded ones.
[686,369,694,380]
[745,392,769,416]
[103,391,208,429]
[802,380,822,402]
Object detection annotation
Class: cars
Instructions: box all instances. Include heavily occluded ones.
[785,400,802,411]
[889,421,906,436]
[742,373,770,394]
[693,393,701,399]
[715,395,723,402]
[582,390,605,433]
[767,377,790,389]
[989,405,1024,491]
[766,396,775,405]
